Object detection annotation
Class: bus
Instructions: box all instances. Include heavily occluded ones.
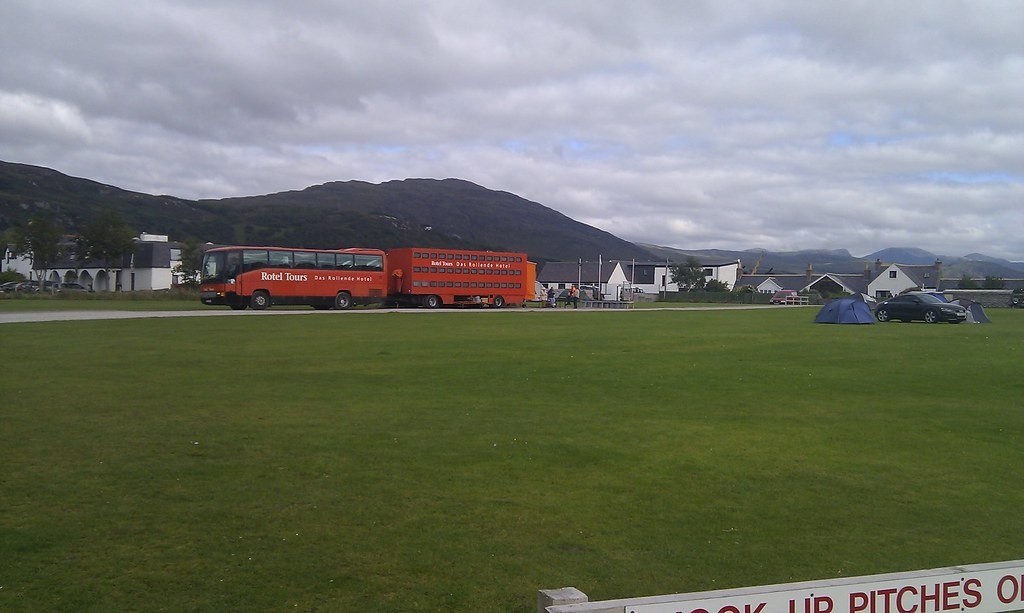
[198,246,389,310]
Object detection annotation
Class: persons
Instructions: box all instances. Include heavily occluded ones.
[540,288,548,308]
[888,293,898,299]
[547,287,555,308]
[565,285,577,305]
[573,286,579,309]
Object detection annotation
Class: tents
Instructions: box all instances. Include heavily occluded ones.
[919,292,949,304]
[813,296,876,324]
[531,281,548,302]
[947,298,992,323]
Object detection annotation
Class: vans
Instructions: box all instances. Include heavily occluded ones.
[769,290,797,305]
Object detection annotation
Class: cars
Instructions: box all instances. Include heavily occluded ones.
[874,293,968,324]
[0,279,89,295]
